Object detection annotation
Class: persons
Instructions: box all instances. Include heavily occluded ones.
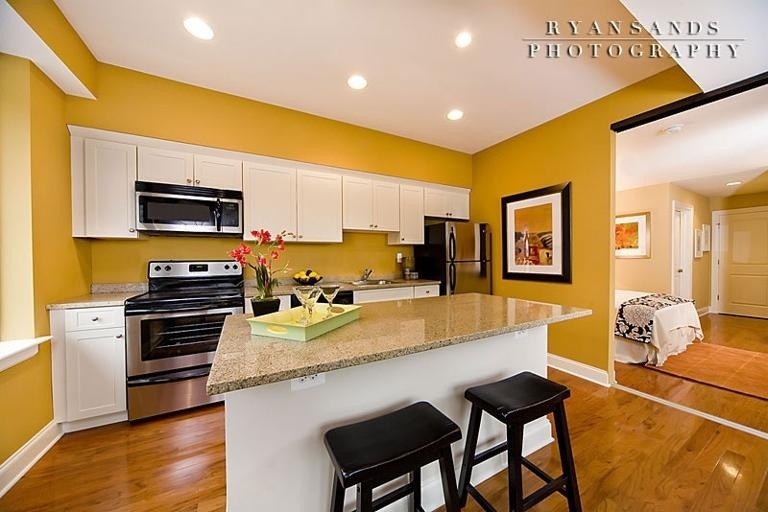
[522,224,531,258]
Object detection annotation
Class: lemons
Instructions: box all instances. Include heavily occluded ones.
[294,270,317,281]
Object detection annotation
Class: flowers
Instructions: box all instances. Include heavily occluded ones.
[227,229,296,299]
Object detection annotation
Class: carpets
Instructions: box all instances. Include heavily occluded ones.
[645,341,768,401]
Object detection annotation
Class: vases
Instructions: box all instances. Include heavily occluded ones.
[250,299,281,318]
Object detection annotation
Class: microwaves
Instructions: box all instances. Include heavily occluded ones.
[134,180,244,237]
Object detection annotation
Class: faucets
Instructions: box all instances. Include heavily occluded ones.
[367,270,372,277]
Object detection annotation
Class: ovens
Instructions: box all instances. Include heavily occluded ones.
[124,305,244,423]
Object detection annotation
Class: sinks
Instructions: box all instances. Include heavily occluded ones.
[352,279,400,284]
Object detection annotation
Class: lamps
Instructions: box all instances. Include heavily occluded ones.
[662,123,685,136]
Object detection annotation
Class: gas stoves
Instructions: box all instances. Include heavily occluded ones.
[124,285,245,307]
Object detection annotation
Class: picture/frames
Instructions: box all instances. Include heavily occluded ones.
[694,224,711,258]
[501,181,571,284]
[614,211,652,259]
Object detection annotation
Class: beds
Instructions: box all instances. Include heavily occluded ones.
[613,289,704,365]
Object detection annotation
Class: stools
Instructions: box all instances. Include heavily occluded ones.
[322,401,463,512]
[458,372,582,512]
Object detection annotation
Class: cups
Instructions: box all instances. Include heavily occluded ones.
[529,233,538,256]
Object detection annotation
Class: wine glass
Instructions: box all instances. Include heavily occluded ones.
[291,284,341,326]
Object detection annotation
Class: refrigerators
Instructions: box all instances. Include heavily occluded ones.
[415,221,492,298]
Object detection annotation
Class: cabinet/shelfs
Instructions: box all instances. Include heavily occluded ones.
[242,153,343,244]
[424,181,472,220]
[344,169,400,234]
[137,135,242,191]
[47,301,130,434]
[388,178,424,246]
[66,123,137,240]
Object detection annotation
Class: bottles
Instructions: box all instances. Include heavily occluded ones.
[524,231,529,258]
